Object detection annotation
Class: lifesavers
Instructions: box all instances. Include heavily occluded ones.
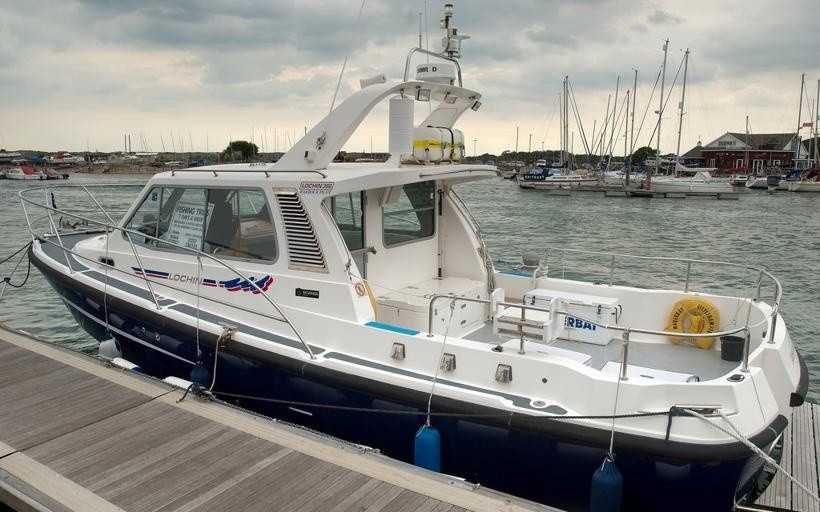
[667,299,720,347]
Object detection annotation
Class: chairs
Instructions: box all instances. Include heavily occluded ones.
[203,189,233,253]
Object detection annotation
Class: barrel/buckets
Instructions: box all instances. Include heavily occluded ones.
[719,324,744,361]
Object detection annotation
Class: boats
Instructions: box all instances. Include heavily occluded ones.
[6,168,69,180]
[26,0,809,510]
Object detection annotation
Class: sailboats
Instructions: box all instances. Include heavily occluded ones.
[496,38,820,197]
[0,127,212,166]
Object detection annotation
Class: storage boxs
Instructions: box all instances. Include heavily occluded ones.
[526,288,618,346]
